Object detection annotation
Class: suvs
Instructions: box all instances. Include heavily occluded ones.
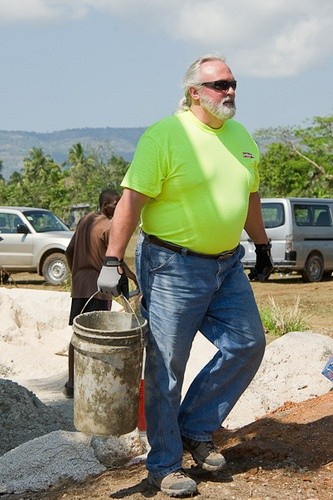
[0,206,77,287]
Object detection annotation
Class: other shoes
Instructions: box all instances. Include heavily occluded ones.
[62,382,74,397]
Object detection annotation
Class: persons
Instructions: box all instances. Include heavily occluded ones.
[98,55,274,497]
[62,189,137,399]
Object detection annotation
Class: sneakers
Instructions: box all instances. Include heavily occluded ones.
[147,469,197,497]
[181,436,227,471]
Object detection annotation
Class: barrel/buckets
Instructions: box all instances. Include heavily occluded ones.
[70,289,147,437]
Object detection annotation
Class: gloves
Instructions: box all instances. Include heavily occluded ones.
[248,243,273,282]
[97,255,129,300]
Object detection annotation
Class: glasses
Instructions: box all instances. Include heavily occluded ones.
[198,79,237,90]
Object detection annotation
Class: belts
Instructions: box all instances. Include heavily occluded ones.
[145,233,241,262]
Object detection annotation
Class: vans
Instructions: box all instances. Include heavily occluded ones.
[239,197,332,281]
